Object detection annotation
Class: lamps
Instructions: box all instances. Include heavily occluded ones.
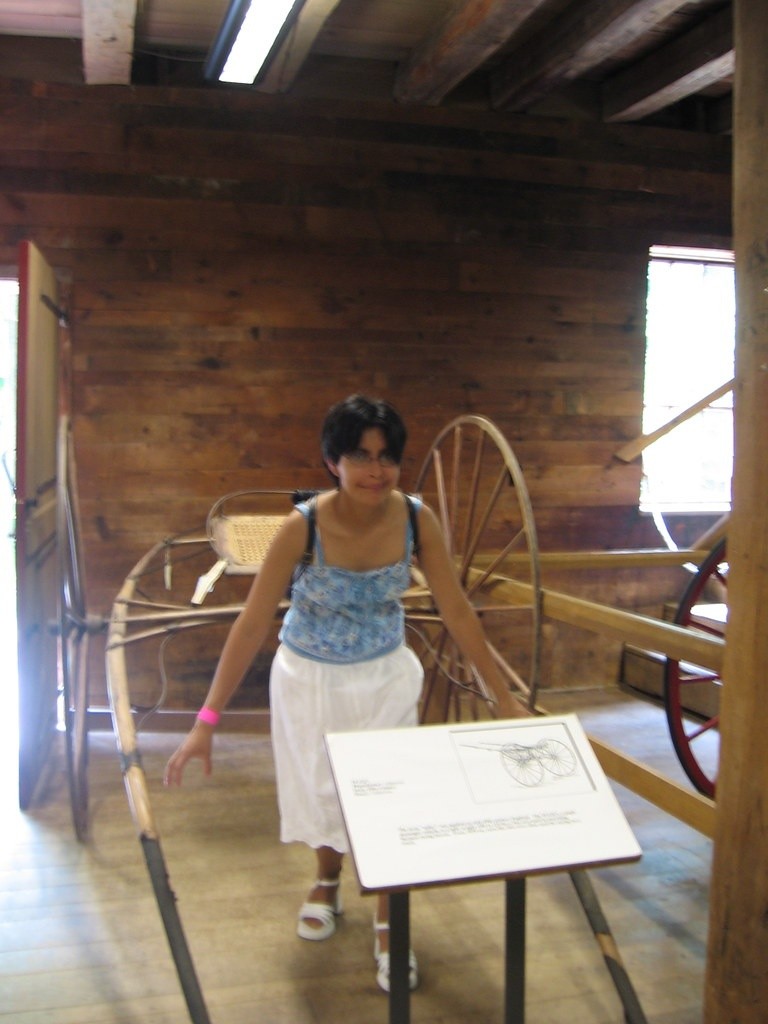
[204,0,304,86]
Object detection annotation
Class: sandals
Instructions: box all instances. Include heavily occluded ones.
[297,877,344,940]
[375,911,417,991]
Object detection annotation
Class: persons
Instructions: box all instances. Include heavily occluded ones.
[162,394,538,993]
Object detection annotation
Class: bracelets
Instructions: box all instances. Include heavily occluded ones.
[197,707,221,725]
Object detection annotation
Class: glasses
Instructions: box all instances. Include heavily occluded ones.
[349,449,399,466]
